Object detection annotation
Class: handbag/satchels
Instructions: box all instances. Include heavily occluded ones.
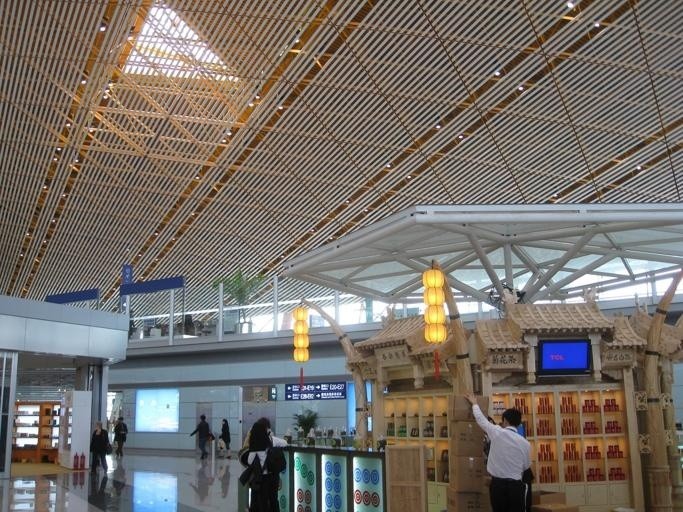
[268,447,285,471]
[208,432,215,441]
[107,444,112,454]
[218,439,224,448]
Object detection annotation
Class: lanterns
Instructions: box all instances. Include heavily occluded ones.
[293,307,309,387]
[422,270,447,382]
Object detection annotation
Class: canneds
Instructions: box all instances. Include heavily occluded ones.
[423,420,434,437]
[494,396,625,483]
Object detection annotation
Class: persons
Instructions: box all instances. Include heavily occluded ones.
[190,460,209,502]
[464,391,532,512]
[220,460,230,498]
[113,456,126,496]
[243,418,288,512]
[90,422,107,473]
[218,420,230,459]
[190,415,209,459]
[114,418,127,456]
[88,472,108,512]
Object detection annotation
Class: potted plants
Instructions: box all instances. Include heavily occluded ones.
[213,269,269,334]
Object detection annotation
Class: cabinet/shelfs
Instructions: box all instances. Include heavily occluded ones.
[13,400,73,452]
[383,382,629,492]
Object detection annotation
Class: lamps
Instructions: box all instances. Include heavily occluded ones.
[423,258,449,344]
[293,300,311,364]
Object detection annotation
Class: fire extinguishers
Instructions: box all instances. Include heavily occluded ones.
[74,452,85,470]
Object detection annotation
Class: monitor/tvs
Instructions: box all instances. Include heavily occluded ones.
[517,424,525,438]
[537,339,591,375]
